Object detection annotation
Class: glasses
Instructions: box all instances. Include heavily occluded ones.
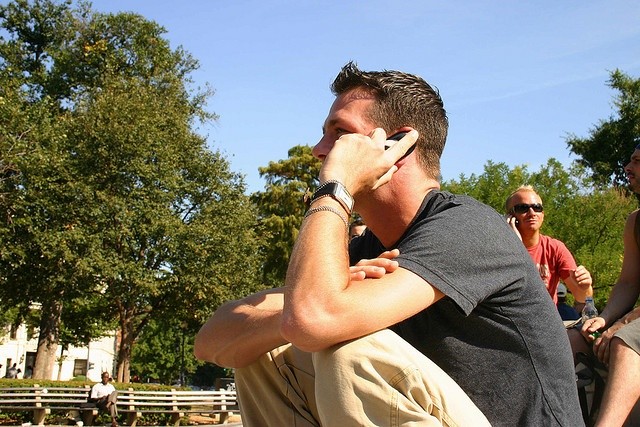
[506,203,544,215]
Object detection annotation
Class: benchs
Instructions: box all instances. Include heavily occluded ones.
[1,382,240,427]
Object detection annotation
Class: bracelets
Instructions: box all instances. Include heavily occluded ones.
[304,179,356,228]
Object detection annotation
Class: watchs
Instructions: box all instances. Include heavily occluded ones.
[313,183,355,215]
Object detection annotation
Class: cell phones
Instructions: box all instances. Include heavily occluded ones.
[509,217,519,226]
[385,131,416,165]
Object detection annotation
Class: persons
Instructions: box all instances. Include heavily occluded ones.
[504,184,594,321]
[8,363,16,378]
[565,142,640,427]
[23,366,33,379]
[0,364,3,368]
[193,61,587,427]
[15,369,21,378]
[90,372,119,427]
